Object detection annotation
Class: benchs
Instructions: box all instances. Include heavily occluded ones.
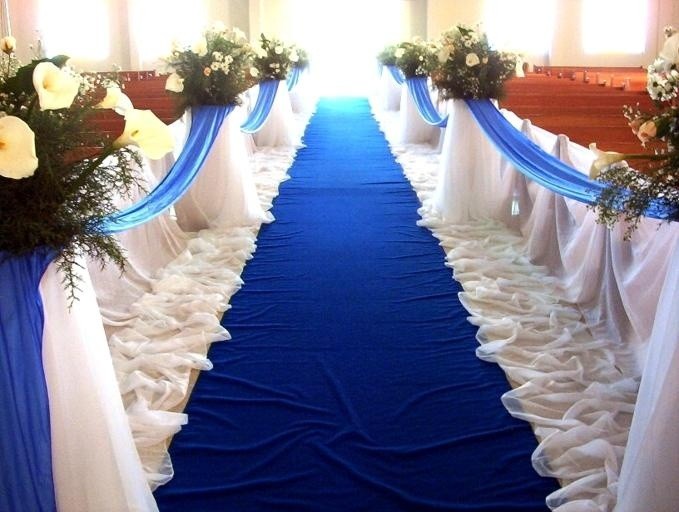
[504,64,667,185]
[66,66,183,165]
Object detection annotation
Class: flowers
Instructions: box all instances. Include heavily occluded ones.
[291,44,311,73]
[251,33,291,86]
[428,21,513,105]
[152,19,268,106]
[581,25,679,243]
[395,37,442,75]
[0,32,182,301]
